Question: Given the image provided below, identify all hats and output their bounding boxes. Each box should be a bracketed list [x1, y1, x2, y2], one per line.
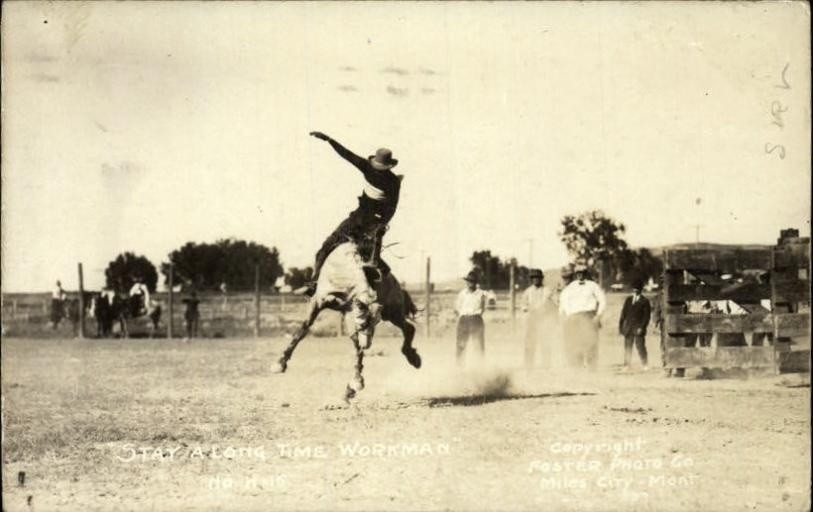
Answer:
[529, 269, 545, 279]
[368, 148, 397, 171]
[632, 278, 642, 289]
[574, 264, 588, 274]
[462, 272, 481, 283]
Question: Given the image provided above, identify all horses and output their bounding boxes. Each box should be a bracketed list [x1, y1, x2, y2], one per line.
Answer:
[268, 235, 425, 400]
[49, 291, 161, 339]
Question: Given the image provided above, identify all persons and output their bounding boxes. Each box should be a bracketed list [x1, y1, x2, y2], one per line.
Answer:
[522, 266, 551, 369]
[558, 264, 606, 369]
[48, 278, 201, 340]
[294, 132, 400, 296]
[617, 279, 650, 366]
[455, 273, 495, 357]
[673, 271, 772, 377]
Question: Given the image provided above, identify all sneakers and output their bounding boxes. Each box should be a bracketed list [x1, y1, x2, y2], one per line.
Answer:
[616, 367, 633, 375]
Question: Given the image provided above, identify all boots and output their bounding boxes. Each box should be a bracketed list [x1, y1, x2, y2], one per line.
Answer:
[293, 271, 318, 296]
